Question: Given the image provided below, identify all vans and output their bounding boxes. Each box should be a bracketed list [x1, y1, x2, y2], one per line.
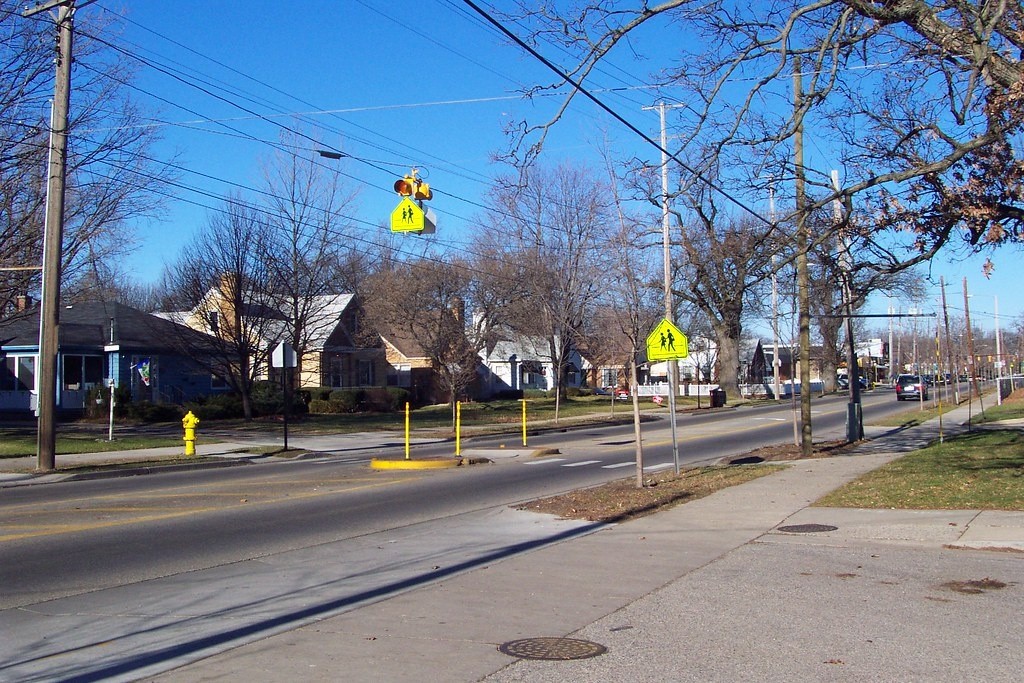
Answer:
[896, 374, 928, 400]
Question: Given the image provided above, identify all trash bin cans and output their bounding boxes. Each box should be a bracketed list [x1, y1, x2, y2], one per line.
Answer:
[710, 390, 727, 408]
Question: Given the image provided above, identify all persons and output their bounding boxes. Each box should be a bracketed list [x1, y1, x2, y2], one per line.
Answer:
[867, 368, 873, 389]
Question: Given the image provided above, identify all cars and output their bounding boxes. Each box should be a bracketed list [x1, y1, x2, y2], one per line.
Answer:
[838, 374, 870, 390]
[924, 372, 987, 385]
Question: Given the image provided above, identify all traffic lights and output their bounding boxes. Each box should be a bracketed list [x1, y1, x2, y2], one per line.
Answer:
[393, 178, 414, 195]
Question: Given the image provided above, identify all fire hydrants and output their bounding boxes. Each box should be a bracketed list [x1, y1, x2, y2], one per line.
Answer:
[181, 411, 201, 456]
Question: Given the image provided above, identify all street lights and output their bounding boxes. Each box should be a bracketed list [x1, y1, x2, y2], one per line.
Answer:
[965, 293, 1002, 405]
